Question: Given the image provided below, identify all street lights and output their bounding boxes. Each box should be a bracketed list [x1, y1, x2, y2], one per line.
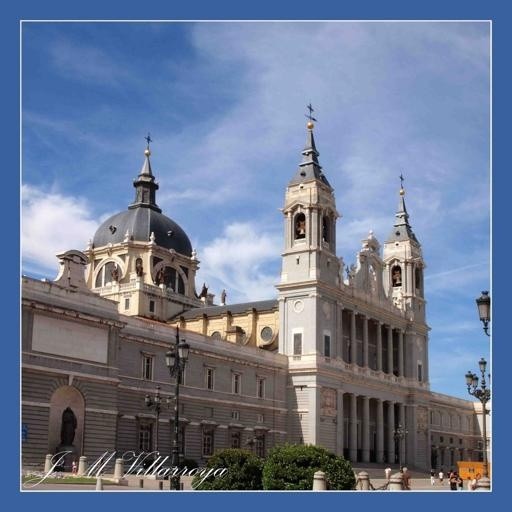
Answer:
[465, 357, 490, 479]
[475, 289, 490, 336]
[166, 325, 189, 490]
[145, 384, 172, 473]
[393, 424, 408, 473]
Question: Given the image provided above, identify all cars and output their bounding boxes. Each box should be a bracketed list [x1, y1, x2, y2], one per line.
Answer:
[124, 455, 165, 475]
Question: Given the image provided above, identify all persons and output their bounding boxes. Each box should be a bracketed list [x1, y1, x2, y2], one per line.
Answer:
[384, 465, 411, 486]
[201, 283, 208, 297]
[110, 266, 119, 281]
[430, 468, 480, 490]
[135, 259, 143, 277]
[221, 290, 226, 303]
[54, 457, 78, 475]
